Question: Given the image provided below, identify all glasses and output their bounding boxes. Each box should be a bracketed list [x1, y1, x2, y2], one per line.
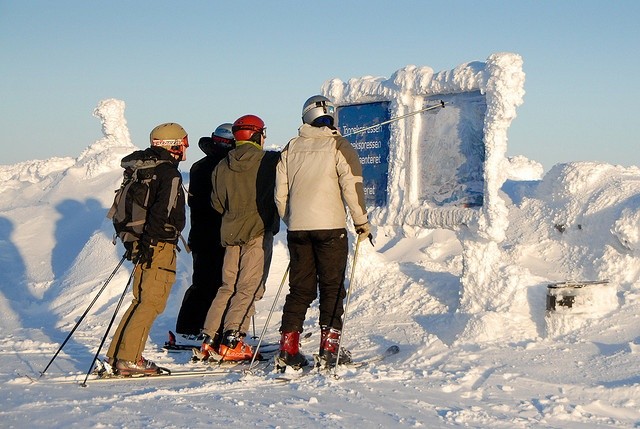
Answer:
[234, 126, 267, 139]
[152, 136, 189, 149]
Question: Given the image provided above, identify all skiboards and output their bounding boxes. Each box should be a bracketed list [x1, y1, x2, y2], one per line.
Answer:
[15, 360, 260, 384]
[235, 345, 400, 388]
[160, 332, 312, 353]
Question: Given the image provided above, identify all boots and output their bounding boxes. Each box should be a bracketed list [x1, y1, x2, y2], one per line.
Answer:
[281, 331, 305, 366]
[319, 326, 351, 362]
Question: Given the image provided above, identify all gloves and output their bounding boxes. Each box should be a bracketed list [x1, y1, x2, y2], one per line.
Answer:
[133, 241, 154, 263]
[354, 223, 370, 242]
[123, 243, 133, 260]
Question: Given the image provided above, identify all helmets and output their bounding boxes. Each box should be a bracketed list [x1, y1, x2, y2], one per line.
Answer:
[302, 95, 334, 124]
[212, 123, 233, 147]
[234, 115, 265, 140]
[149, 123, 189, 161]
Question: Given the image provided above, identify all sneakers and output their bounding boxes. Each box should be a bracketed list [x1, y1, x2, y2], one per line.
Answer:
[116, 357, 160, 375]
[219, 341, 263, 361]
[199, 336, 221, 358]
[175, 333, 205, 347]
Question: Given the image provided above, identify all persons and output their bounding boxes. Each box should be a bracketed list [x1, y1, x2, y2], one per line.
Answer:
[101, 122, 190, 373]
[173, 122, 237, 348]
[200, 114, 282, 359]
[273, 95, 371, 372]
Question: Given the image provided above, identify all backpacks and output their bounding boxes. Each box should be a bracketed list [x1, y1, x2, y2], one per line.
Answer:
[107, 150, 174, 242]
